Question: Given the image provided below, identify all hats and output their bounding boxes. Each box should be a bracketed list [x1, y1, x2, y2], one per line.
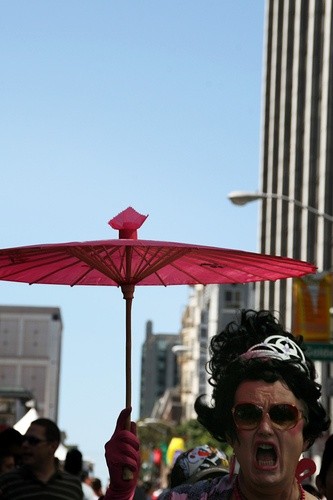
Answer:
[171, 446, 230, 486]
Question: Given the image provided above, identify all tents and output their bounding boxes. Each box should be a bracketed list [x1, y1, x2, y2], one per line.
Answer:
[13, 408, 68, 462]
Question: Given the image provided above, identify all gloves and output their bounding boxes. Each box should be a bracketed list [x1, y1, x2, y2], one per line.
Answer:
[104, 407, 141, 500]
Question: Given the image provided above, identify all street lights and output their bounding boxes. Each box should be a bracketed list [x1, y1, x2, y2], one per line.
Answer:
[171, 345, 189, 425]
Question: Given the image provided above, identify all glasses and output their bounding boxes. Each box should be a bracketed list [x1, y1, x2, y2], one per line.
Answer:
[232, 403, 305, 431]
[20, 435, 49, 447]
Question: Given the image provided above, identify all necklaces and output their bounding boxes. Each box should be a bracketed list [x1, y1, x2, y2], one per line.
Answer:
[234, 480, 305, 500]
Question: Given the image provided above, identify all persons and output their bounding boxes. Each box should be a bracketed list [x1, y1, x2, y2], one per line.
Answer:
[168, 444, 230, 489]
[101, 309, 332, 500]
[91, 478, 104, 497]
[315, 434, 333, 500]
[64, 446, 100, 500]
[0, 418, 85, 500]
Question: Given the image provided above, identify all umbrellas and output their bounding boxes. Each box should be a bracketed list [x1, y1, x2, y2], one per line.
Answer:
[0, 206, 318, 482]
